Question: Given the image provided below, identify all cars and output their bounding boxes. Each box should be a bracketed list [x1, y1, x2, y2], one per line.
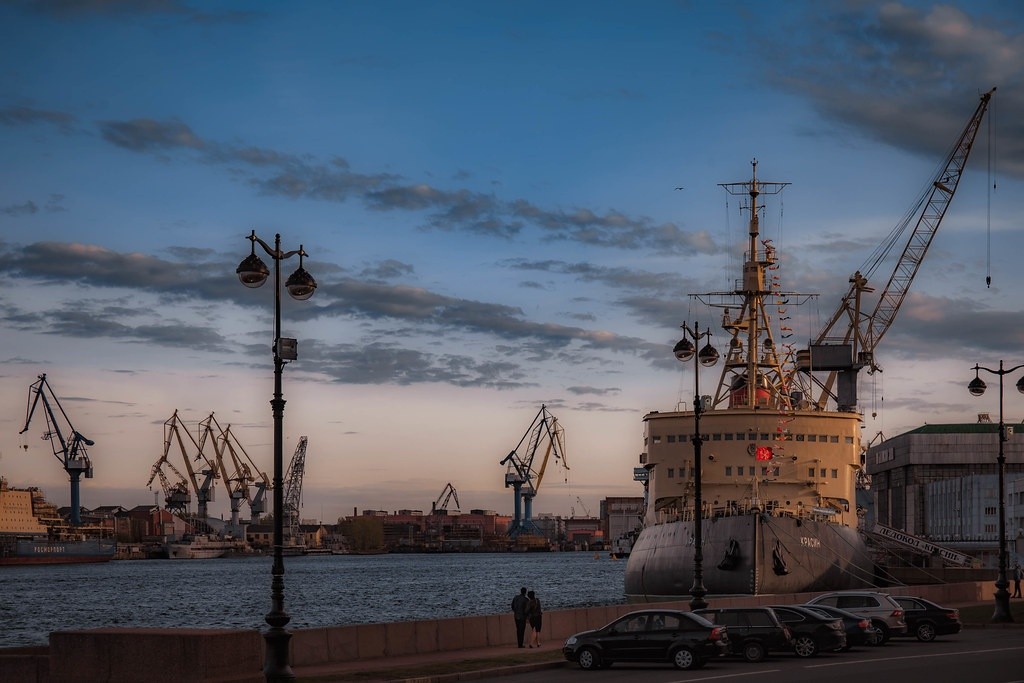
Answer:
[795, 604, 877, 654]
[563, 609, 732, 670]
[890, 594, 962, 643]
[763, 604, 848, 658]
[689, 607, 791, 662]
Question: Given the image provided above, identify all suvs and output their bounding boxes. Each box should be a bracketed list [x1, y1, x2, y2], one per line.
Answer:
[805, 592, 908, 646]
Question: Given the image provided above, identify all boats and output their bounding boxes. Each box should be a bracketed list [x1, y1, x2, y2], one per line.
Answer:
[268, 545, 309, 558]
[0, 528, 118, 565]
[619, 150, 886, 600]
[329, 547, 351, 556]
[609, 537, 633, 557]
[167, 534, 245, 559]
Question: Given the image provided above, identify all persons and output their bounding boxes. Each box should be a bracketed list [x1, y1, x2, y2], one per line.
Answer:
[524, 591, 543, 648]
[626, 617, 646, 632]
[511, 587, 529, 647]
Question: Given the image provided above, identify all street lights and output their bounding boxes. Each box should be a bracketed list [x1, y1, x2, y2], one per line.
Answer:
[968, 355, 1024, 624]
[233, 228, 318, 682]
[671, 317, 722, 614]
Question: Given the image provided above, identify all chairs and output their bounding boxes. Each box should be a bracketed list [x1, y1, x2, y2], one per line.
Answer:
[647, 621, 658, 630]
[656, 619, 664, 629]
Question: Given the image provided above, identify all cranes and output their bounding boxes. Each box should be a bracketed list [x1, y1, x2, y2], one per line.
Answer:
[145, 406, 316, 549]
[499, 400, 577, 552]
[421, 482, 461, 554]
[818, 87, 1005, 587]
[17, 370, 96, 530]
